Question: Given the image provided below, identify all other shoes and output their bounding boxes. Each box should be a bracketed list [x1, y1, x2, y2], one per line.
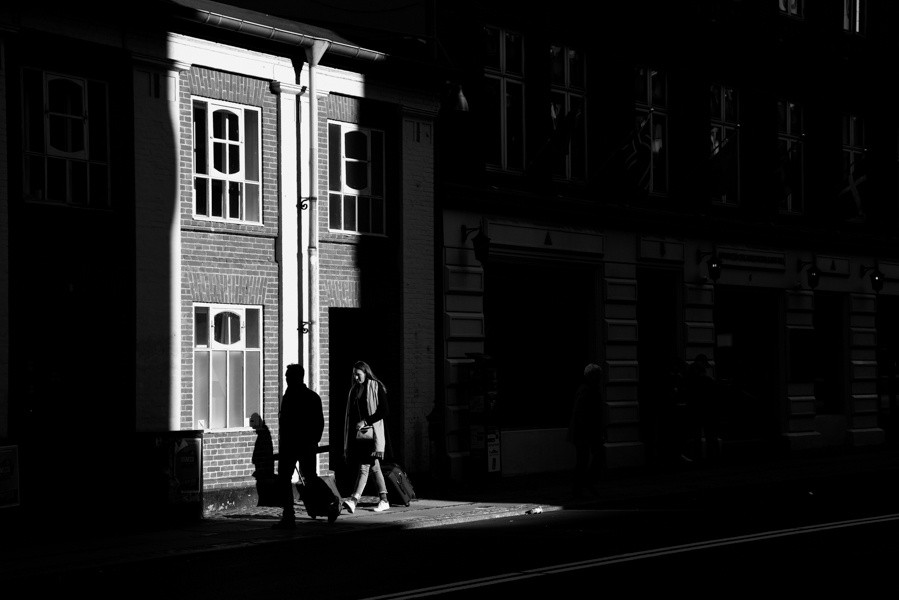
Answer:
[344, 500, 355, 513]
[374, 501, 390, 511]
[271, 520, 297, 529]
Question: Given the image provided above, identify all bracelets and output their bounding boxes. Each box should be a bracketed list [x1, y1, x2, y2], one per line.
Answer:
[364, 420, 368, 426]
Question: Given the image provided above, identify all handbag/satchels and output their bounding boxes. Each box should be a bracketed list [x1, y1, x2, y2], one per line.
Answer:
[356, 426, 374, 440]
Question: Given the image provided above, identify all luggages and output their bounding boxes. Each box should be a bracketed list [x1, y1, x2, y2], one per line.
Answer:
[372, 464, 416, 506]
[295, 466, 343, 519]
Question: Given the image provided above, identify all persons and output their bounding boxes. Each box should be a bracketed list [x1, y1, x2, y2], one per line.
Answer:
[570, 363, 608, 489]
[343, 361, 390, 514]
[679, 354, 725, 462]
[271, 364, 341, 530]
